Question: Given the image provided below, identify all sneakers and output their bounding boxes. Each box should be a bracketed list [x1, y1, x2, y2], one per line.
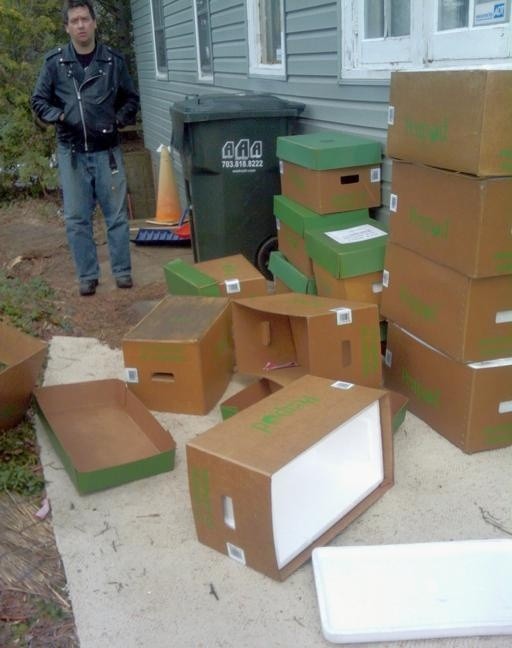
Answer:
[116, 275, 133, 288]
[80, 279, 99, 296]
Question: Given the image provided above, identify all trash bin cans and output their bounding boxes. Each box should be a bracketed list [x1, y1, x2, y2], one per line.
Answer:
[170, 90, 306, 282]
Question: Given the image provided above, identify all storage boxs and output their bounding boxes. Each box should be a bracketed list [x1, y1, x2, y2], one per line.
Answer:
[222, 70, 512, 456]
[2, 322, 50, 428]
[122, 297, 232, 416]
[165, 254, 266, 298]
[186, 374, 396, 581]
[34, 377, 177, 496]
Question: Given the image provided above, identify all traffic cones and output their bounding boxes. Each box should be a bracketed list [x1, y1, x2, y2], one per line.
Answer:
[144, 145, 189, 226]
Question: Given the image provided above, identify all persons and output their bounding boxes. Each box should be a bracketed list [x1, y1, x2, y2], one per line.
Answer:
[30, 0, 140, 295]
